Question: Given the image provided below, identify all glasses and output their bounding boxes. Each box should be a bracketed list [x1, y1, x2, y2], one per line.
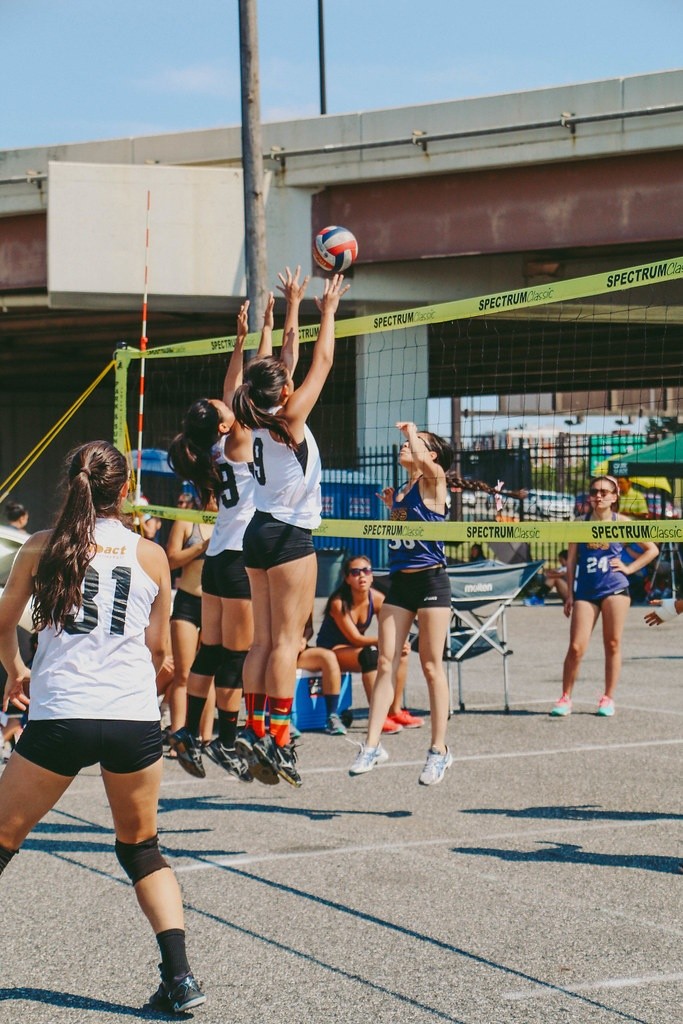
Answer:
[177, 501, 186, 505]
[590, 489, 612, 497]
[407, 436, 432, 452]
[348, 567, 372, 576]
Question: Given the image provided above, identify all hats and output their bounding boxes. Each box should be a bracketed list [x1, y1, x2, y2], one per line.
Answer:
[131, 496, 151, 525]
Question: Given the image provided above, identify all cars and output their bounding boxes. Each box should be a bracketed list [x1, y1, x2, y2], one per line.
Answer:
[645, 494, 679, 520]
[458, 490, 476, 508]
[574, 494, 590, 513]
[487, 490, 575, 518]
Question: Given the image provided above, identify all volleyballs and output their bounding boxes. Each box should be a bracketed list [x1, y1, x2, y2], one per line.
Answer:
[313, 225, 358, 273]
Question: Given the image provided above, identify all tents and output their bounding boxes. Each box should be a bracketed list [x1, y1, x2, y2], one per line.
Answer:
[606, 432, 683, 480]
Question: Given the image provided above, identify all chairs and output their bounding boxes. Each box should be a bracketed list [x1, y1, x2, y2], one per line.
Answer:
[372, 559, 547, 718]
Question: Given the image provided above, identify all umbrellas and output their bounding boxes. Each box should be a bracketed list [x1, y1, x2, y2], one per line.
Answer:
[595, 453, 672, 492]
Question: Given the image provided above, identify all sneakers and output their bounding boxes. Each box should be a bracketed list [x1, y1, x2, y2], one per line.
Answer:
[234, 728, 280, 785]
[148, 962, 207, 1012]
[552, 692, 572, 716]
[289, 722, 301, 739]
[381, 717, 403, 734]
[344, 737, 389, 775]
[168, 727, 206, 778]
[387, 710, 425, 729]
[253, 734, 303, 788]
[597, 695, 615, 716]
[203, 737, 254, 783]
[418, 744, 453, 787]
[325, 713, 347, 735]
[523, 595, 544, 607]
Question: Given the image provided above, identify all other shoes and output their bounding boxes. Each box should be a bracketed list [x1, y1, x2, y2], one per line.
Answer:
[162, 723, 172, 743]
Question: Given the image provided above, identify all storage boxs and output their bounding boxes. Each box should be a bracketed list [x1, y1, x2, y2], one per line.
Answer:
[290, 668, 352, 735]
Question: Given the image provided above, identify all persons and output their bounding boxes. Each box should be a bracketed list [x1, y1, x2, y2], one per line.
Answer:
[469, 544, 485, 562]
[0, 265, 348, 788]
[349, 422, 454, 785]
[521, 497, 683, 627]
[546, 481, 660, 717]
[0, 439, 209, 1013]
[320, 555, 425, 734]
